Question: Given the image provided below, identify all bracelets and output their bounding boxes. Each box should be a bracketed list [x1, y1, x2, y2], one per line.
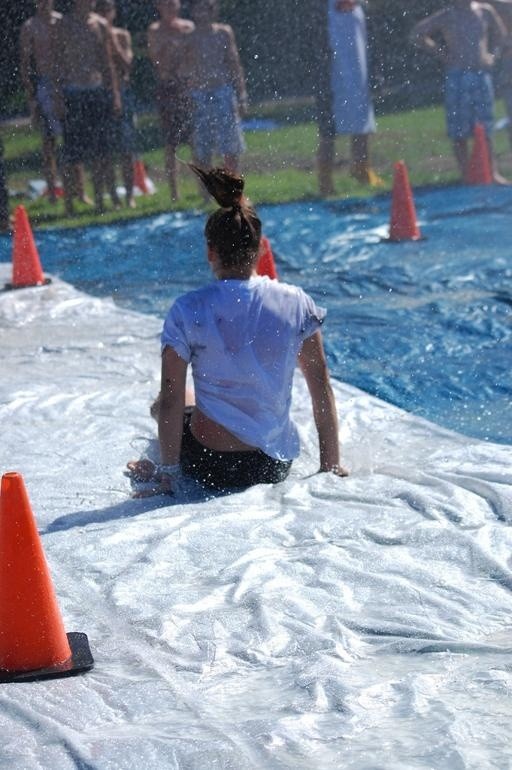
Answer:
[158, 463, 183, 475]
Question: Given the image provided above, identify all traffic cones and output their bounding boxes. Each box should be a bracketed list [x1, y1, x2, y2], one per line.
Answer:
[4, 203, 54, 290]
[0, 468, 95, 686]
[457, 124, 492, 185]
[378, 160, 429, 242]
[255, 234, 277, 280]
[40, 157, 64, 198]
[135, 161, 150, 196]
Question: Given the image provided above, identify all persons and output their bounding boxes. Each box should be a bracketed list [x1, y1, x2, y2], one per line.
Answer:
[18, 0, 137, 217]
[327, 0, 385, 187]
[126, 156, 350, 498]
[146, 0, 249, 210]
[409, 0, 512, 186]
